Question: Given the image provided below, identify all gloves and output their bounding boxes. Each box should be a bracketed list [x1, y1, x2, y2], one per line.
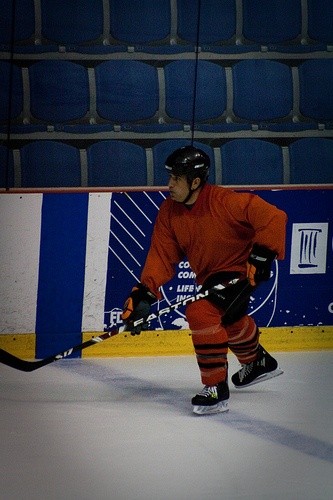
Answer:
[245, 242, 277, 286]
[121, 283, 158, 336]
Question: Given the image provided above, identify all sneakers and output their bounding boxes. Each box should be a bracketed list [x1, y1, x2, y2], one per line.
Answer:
[192, 380, 231, 415]
[231, 345, 284, 388]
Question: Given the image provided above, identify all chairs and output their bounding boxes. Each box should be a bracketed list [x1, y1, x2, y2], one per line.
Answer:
[0, 0, 333, 187]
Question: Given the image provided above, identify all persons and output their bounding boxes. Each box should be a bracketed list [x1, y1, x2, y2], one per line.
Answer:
[122, 145, 287, 406]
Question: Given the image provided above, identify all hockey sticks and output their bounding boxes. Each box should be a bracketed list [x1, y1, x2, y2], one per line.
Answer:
[0, 269, 276, 372]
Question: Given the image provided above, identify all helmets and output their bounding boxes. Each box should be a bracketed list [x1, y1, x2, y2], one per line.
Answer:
[166, 145, 211, 184]
